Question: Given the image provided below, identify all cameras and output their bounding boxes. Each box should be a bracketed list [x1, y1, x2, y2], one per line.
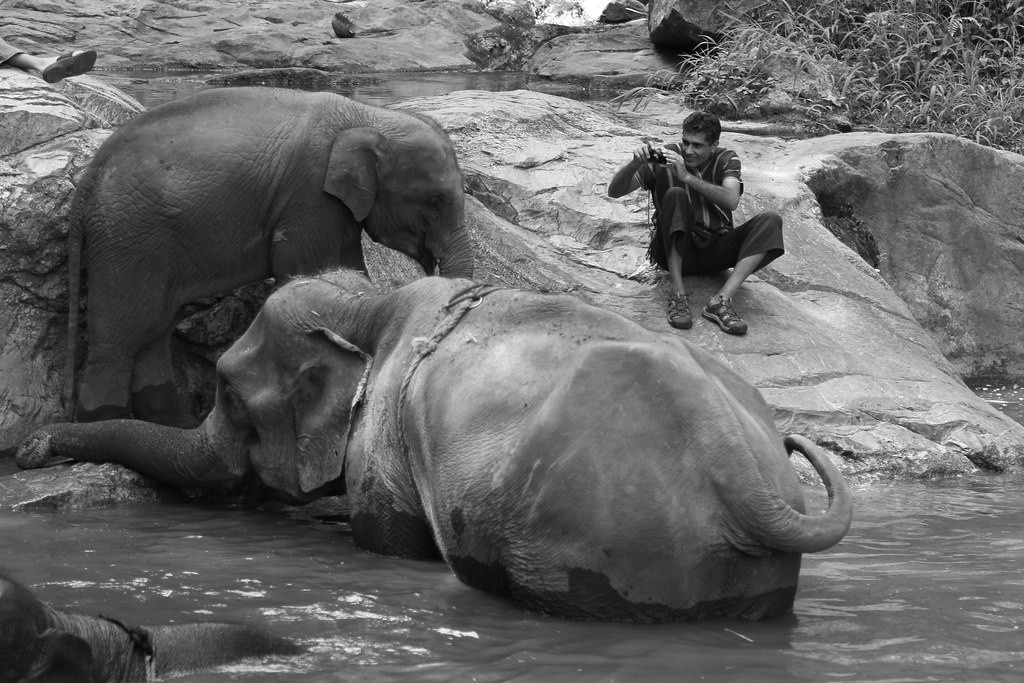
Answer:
[646, 143, 682, 164]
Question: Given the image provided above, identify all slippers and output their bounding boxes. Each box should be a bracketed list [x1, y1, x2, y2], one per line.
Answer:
[43, 49, 97, 83]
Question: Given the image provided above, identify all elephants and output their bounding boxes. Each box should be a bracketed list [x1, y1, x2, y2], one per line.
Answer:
[17, 85, 855, 624]
[0, 569, 310, 683]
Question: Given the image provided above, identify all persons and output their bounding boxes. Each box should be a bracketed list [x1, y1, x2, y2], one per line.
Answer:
[608, 112, 785, 336]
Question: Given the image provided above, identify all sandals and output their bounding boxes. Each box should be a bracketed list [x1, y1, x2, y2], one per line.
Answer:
[701, 296, 747, 334]
[667, 292, 692, 329]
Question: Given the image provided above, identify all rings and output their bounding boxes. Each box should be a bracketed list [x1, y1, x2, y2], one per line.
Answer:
[676, 157, 679, 160]
